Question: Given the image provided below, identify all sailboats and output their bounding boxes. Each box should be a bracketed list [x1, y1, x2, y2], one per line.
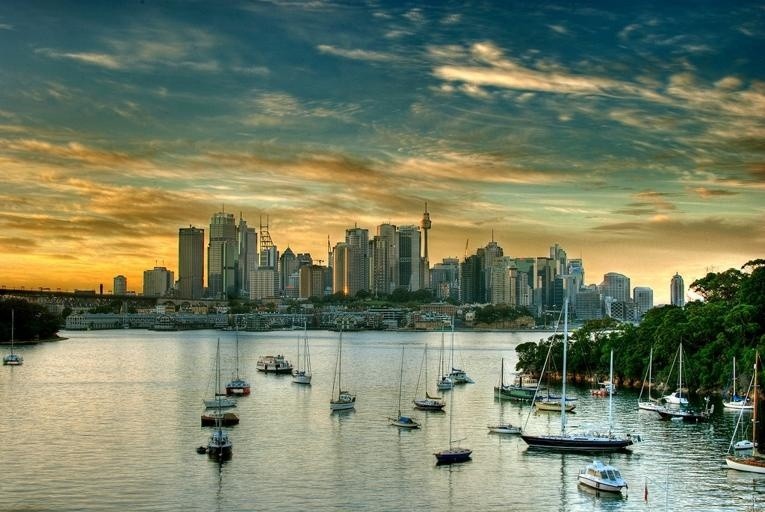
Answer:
[3, 309, 23, 365]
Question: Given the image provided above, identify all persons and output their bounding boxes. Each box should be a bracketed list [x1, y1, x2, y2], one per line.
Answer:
[600, 385, 607, 391]
[756, 387, 765, 455]
